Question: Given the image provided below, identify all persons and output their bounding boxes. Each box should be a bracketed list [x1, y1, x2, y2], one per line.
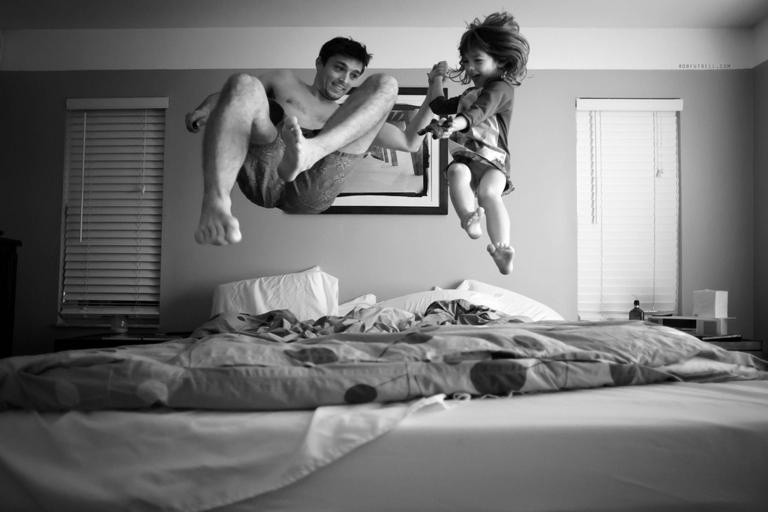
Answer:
[184, 36, 450, 245]
[428, 13, 530, 275]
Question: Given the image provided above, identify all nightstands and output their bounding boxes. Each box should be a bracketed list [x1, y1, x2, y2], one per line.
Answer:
[648, 316, 764, 352]
[93, 322, 182, 347]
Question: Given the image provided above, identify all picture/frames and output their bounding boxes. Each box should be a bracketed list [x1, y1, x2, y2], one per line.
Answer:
[326, 85, 449, 220]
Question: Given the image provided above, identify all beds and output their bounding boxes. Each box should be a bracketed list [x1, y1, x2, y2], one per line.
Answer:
[0, 317, 768, 512]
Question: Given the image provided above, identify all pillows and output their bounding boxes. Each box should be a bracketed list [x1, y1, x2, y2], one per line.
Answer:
[212, 270, 341, 338]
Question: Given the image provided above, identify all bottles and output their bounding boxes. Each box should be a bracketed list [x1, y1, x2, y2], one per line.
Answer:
[629, 300, 644, 322]
